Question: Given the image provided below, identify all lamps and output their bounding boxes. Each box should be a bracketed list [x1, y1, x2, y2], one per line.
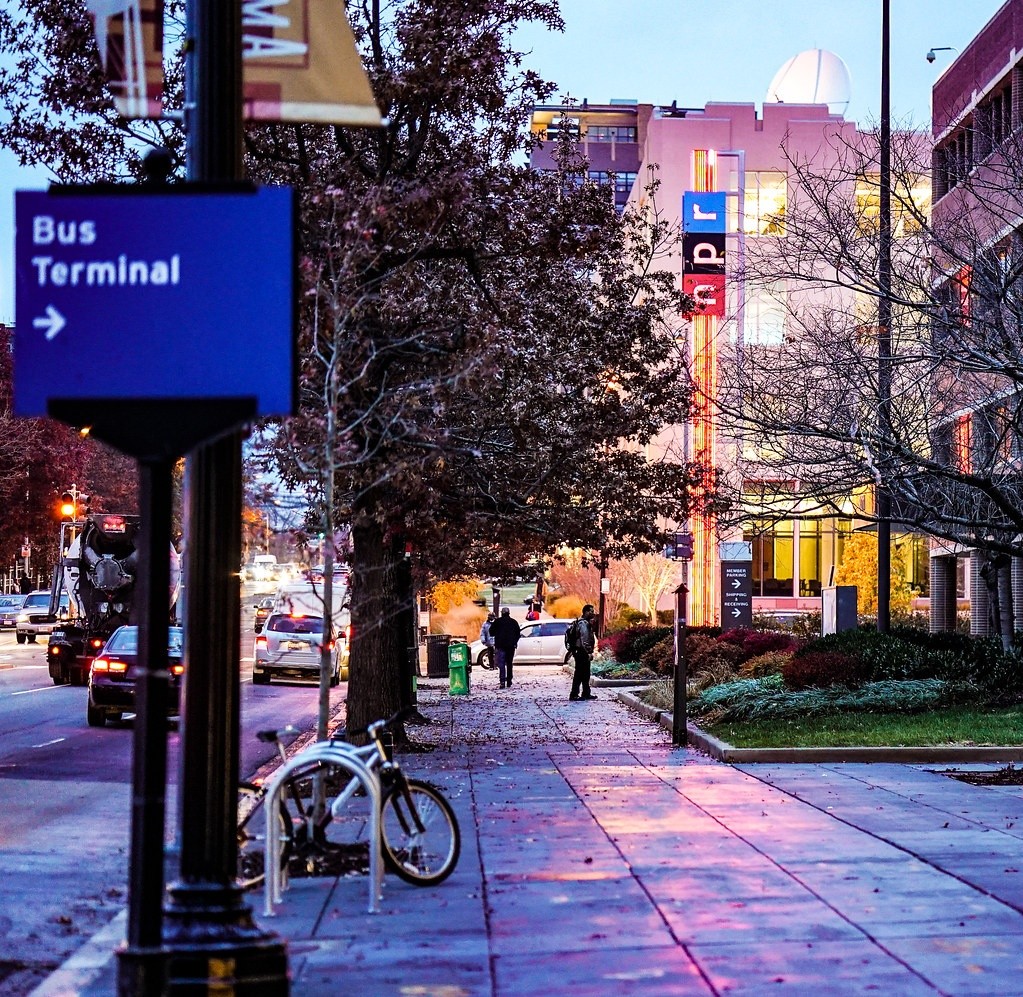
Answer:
[927, 47, 960, 63]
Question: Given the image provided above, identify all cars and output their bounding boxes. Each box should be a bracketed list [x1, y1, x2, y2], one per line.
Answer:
[469, 619, 598, 669]
[0, 593, 30, 629]
[250, 612, 347, 687]
[243, 554, 353, 668]
[86, 624, 184, 728]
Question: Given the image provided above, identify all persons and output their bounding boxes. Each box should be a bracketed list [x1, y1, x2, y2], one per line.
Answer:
[520, 626, 540, 637]
[569, 605, 598, 701]
[481, 612, 497, 670]
[528, 597, 542, 620]
[13, 570, 31, 594]
[489, 608, 520, 689]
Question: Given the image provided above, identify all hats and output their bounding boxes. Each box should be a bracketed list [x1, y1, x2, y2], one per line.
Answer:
[486, 612, 495, 620]
[501, 608, 510, 614]
[532, 596, 540, 604]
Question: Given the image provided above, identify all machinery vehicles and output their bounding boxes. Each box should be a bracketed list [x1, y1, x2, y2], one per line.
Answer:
[44, 513, 185, 688]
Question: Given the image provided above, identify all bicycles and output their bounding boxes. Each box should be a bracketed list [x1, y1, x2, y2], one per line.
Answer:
[236, 707, 462, 888]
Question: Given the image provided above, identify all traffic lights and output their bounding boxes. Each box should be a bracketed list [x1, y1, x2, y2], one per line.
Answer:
[60, 491, 75, 516]
[77, 490, 91, 516]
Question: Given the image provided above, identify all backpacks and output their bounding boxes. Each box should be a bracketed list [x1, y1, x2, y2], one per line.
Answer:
[564, 618, 589, 654]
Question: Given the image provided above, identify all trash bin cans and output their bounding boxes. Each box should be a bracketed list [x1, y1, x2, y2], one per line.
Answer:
[422, 633, 452, 679]
[447, 640, 473, 695]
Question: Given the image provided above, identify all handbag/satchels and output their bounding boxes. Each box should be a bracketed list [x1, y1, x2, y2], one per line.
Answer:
[525, 612, 535, 621]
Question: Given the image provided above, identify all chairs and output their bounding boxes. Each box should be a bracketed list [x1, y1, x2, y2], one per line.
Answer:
[125, 642, 137, 648]
[276, 621, 295, 630]
[266, 601, 272, 606]
[298, 622, 317, 631]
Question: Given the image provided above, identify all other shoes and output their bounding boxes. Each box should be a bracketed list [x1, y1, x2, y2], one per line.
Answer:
[500, 683, 505, 689]
[581, 694, 597, 699]
[494, 667, 498, 670]
[489, 667, 493, 671]
[507, 681, 512, 687]
[570, 695, 585, 701]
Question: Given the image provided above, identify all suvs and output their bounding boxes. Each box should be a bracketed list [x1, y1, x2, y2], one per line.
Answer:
[14, 587, 75, 644]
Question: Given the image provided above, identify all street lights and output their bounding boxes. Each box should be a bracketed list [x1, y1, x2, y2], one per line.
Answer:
[267, 499, 281, 555]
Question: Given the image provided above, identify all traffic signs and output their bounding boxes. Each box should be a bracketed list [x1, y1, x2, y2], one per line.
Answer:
[5, 182, 302, 424]
